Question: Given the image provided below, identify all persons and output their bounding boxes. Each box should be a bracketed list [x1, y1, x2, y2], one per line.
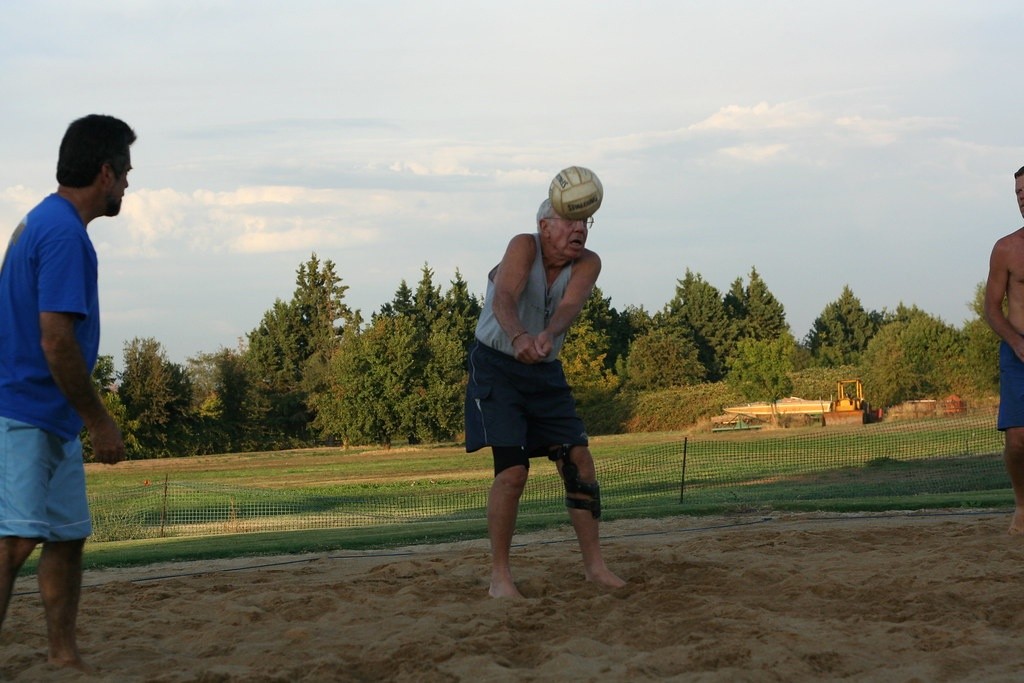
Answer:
[0, 114, 138, 676]
[464, 199, 626, 599]
[984, 166, 1024, 536]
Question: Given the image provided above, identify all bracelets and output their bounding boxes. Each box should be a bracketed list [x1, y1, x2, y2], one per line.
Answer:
[511, 330, 529, 346]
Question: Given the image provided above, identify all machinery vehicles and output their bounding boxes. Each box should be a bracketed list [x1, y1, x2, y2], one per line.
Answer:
[822, 379, 872, 426]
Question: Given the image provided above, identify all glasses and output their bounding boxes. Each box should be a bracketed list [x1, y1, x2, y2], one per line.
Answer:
[543, 216, 594, 228]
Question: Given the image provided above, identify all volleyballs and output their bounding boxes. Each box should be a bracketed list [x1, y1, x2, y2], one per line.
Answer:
[547, 165, 605, 219]
